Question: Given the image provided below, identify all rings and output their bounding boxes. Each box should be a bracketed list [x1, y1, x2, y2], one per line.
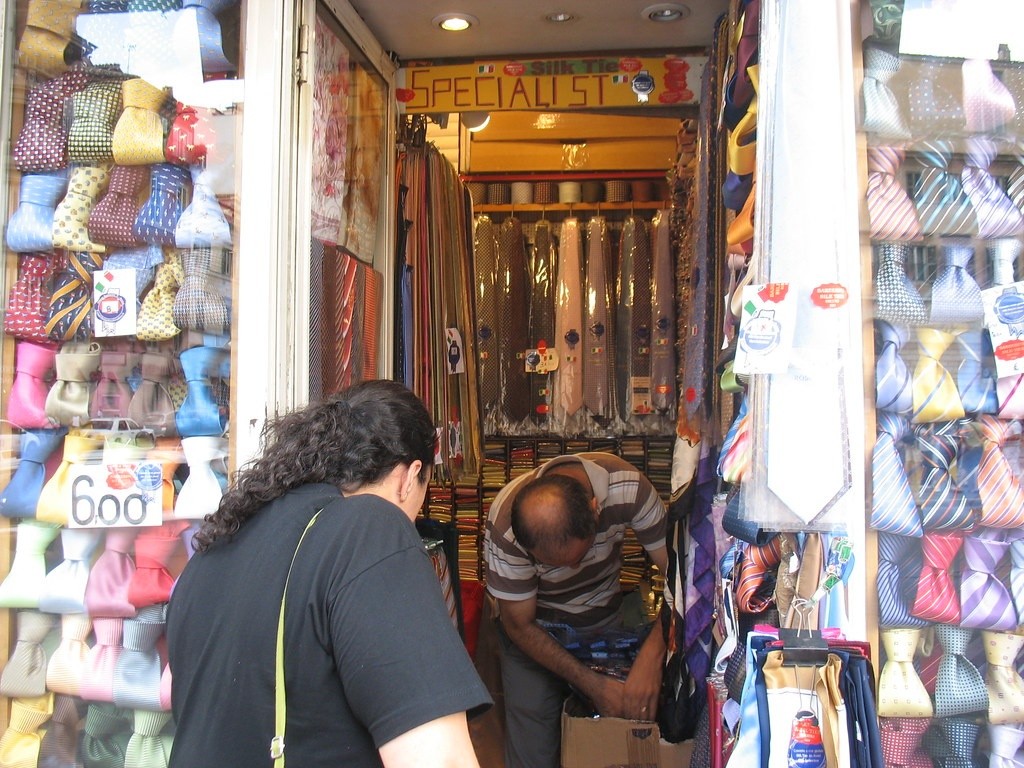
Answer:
[640, 706, 648, 712]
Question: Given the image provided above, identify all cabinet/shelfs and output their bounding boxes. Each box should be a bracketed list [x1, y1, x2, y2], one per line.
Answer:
[415, 171, 676, 602]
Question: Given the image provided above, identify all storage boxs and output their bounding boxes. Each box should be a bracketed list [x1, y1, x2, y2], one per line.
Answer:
[559, 688, 694, 768]
[469, 111, 685, 173]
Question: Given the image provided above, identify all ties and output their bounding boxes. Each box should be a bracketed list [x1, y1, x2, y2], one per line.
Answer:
[399, 147, 466, 474]
[310, 235, 381, 400]
[715, 0, 852, 739]
[862, 48, 1024, 768]
[0, 1, 233, 766]
[473, 180, 676, 439]
[682, 58, 714, 421]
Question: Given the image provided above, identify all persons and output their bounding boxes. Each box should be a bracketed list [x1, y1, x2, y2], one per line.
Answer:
[482, 448, 678, 768]
[160, 379, 496, 768]
